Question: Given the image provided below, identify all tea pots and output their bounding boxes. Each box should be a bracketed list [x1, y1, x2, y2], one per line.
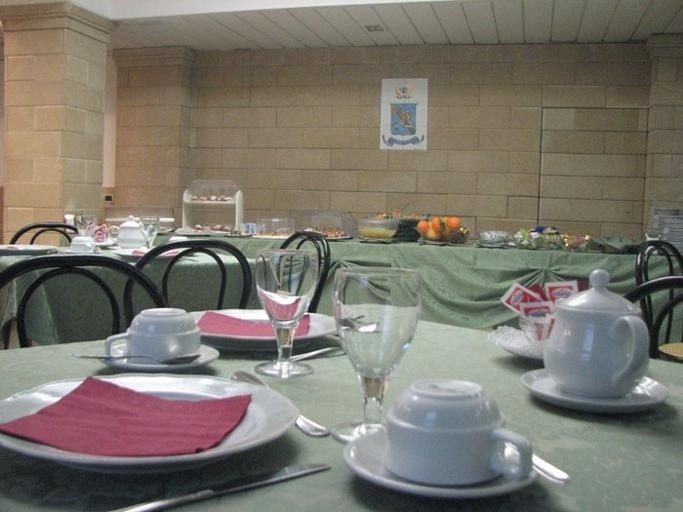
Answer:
[117, 215, 153, 250]
[541, 269, 650, 400]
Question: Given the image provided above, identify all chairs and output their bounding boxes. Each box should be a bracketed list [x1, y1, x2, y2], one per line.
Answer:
[276, 230, 331, 313]
[0, 254, 168, 348]
[8, 221, 80, 246]
[635, 239, 683, 363]
[622, 275, 683, 363]
[123, 238, 252, 329]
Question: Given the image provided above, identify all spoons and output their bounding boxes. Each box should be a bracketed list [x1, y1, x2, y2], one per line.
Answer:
[70, 352, 200, 365]
[289, 322, 381, 364]
[231, 371, 331, 437]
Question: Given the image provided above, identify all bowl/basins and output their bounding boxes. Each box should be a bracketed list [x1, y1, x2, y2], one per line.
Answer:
[519, 315, 546, 347]
[391, 216, 423, 242]
[357, 217, 397, 239]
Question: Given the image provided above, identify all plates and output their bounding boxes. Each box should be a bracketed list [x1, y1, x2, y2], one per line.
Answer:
[0, 372, 299, 473]
[190, 308, 337, 352]
[417, 237, 465, 248]
[341, 433, 539, 500]
[487, 324, 547, 362]
[113, 251, 185, 262]
[252, 234, 291, 241]
[95, 345, 220, 375]
[474, 240, 505, 248]
[325, 236, 350, 243]
[519, 367, 669, 413]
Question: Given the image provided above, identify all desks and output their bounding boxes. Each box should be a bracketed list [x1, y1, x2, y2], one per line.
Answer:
[0, 319, 682, 512]
[105, 224, 683, 332]
[1, 243, 272, 350]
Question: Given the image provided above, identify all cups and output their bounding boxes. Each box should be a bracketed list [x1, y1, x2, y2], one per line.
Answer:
[253, 250, 321, 379]
[103, 307, 201, 364]
[332, 267, 421, 447]
[69, 236, 95, 251]
[383, 378, 534, 485]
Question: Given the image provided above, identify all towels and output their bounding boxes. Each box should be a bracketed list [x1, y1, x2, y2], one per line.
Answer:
[0, 371, 252, 455]
[197, 309, 311, 338]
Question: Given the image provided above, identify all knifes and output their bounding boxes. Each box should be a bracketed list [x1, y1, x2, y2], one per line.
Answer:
[96, 460, 331, 512]
